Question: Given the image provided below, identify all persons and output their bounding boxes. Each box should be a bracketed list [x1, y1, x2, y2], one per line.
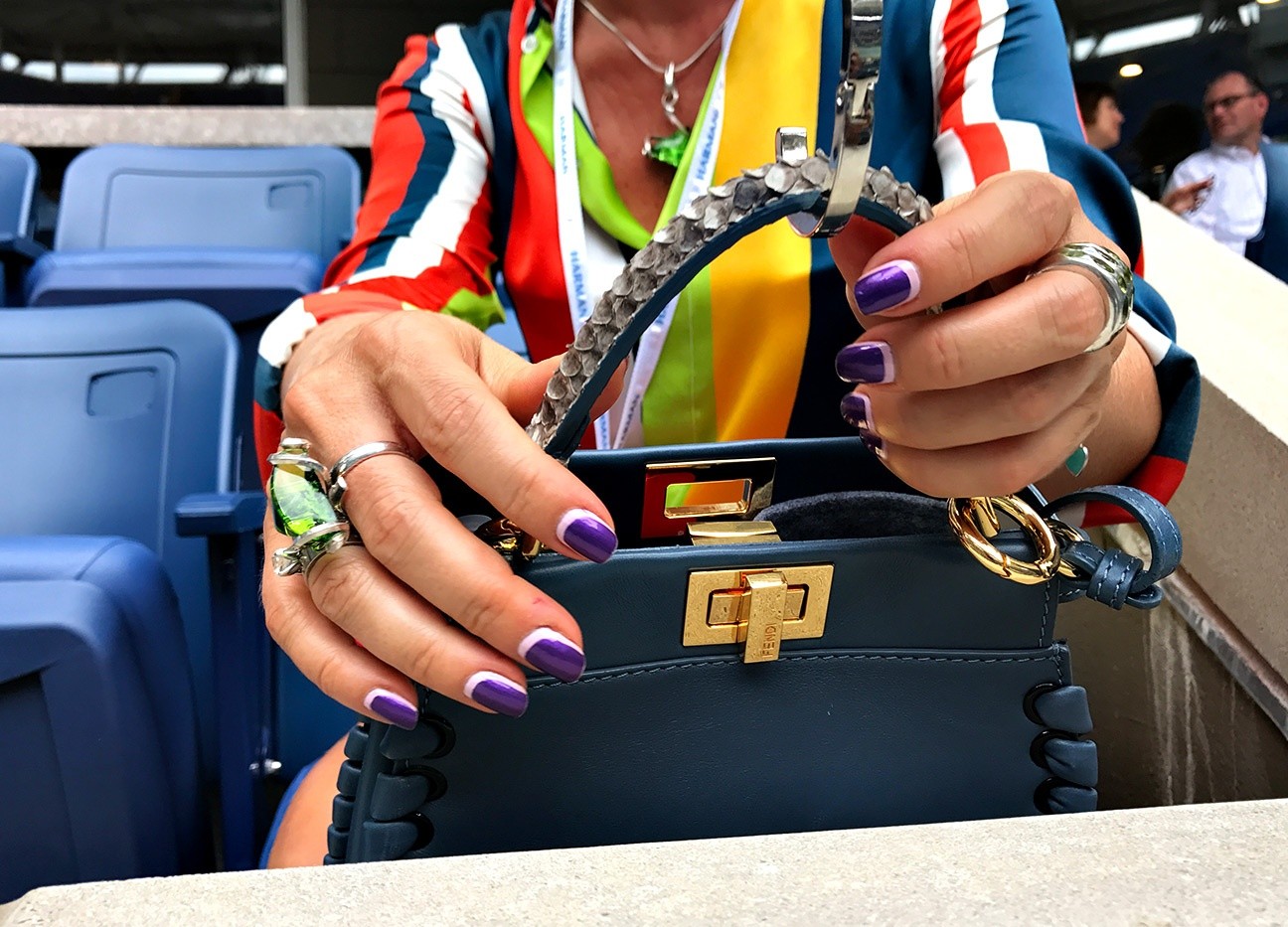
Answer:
[259, 0, 1200, 870]
[1077, 69, 1288, 286]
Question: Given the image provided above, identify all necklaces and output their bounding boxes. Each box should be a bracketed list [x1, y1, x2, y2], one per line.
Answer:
[581, 0, 726, 187]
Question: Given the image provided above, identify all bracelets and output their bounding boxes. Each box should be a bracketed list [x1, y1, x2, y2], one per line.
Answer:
[1066, 445, 1088, 477]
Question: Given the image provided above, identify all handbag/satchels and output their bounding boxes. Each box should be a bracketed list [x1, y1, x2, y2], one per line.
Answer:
[319, 77, 1182, 861]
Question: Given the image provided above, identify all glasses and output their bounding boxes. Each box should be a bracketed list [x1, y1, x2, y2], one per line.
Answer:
[1196, 91, 1259, 114]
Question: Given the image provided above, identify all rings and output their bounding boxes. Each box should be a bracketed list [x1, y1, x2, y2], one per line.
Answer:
[267, 437, 421, 589]
[1025, 243, 1133, 352]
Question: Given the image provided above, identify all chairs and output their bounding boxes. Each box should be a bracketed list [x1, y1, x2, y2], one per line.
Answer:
[0, 141, 361, 903]
